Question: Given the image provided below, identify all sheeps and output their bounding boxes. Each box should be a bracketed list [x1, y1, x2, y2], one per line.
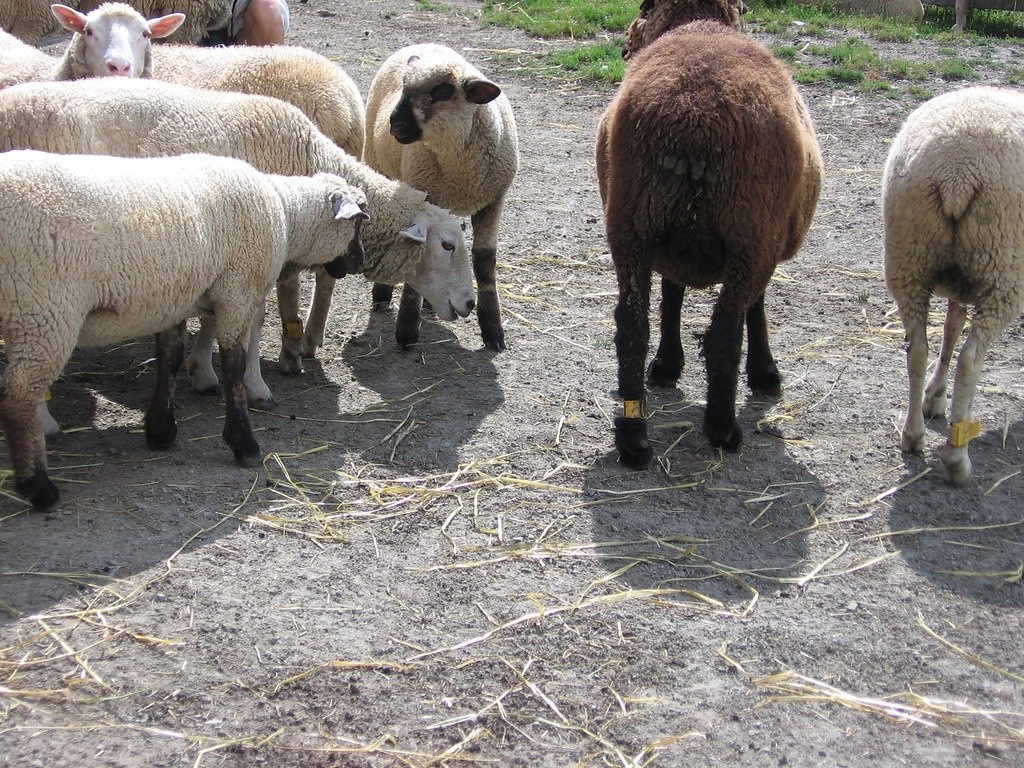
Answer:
[363, 41, 521, 356]
[879, 83, 1024, 491]
[593, 0, 826, 471]
[0, 0, 480, 514]
